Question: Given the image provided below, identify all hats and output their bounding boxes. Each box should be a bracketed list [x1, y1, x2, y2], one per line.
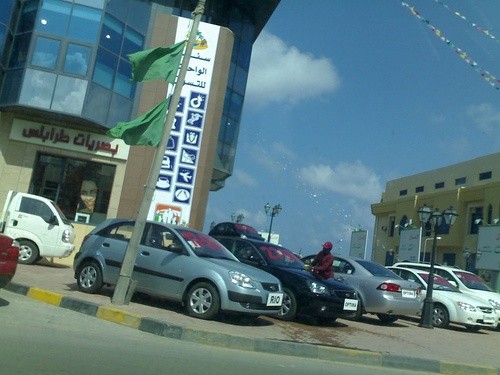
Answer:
[321, 241, 332, 249]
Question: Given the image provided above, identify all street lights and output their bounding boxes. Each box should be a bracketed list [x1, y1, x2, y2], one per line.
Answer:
[264, 202, 283, 241]
[418, 204, 460, 326]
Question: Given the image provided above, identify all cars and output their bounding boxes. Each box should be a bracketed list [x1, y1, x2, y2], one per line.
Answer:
[301, 254, 424, 324]
[208, 222, 264, 240]
[72, 217, 286, 320]
[385, 266, 498, 331]
[212, 235, 364, 325]
[0, 234, 21, 277]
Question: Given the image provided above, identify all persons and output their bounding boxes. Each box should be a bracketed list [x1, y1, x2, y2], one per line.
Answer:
[309, 241, 335, 279]
[80, 180, 98, 212]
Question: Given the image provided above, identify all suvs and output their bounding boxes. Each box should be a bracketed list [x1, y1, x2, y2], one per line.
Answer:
[391, 261, 500, 323]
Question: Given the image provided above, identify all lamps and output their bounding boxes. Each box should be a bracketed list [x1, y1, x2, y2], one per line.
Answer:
[405, 223, 409, 228]
[474, 218, 488, 225]
[395, 224, 404, 230]
[493, 218, 499, 224]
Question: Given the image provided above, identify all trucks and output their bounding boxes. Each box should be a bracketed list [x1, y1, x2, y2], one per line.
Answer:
[0, 189, 77, 266]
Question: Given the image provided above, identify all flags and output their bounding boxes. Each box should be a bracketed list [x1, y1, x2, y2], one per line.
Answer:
[127, 40, 186, 86]
[106, 96, 172, 148]
